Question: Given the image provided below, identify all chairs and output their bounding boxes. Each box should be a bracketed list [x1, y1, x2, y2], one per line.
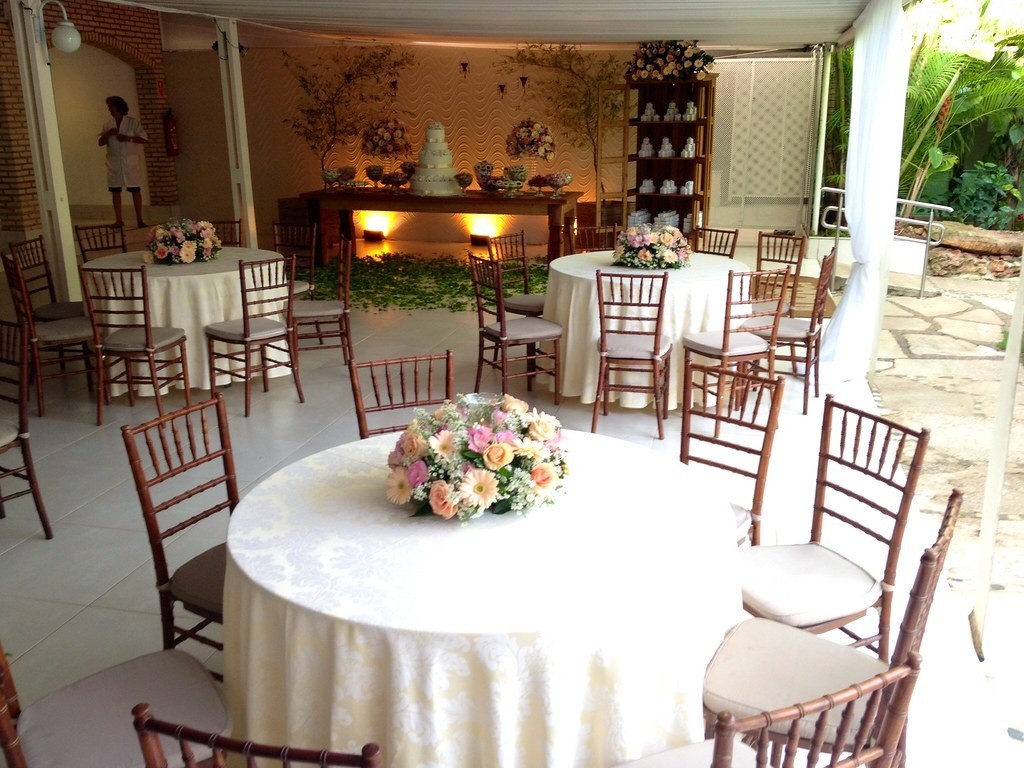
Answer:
[0, 221, 966, 768]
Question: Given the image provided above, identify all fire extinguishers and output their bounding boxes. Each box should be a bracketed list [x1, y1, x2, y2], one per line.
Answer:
[165, 108, 179, 156]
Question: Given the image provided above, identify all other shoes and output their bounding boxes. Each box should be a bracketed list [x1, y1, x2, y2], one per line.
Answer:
[109, 223, 125, 229]
[138, 222, 148, 228]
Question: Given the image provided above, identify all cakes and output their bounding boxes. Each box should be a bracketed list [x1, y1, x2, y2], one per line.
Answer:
[410, 122, 461, 194]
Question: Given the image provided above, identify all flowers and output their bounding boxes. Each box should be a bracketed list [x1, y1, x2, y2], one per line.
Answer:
[143, 218, 222, 264]
[501, 116, 558, 162]
[383, 387, 573, 526]
[361, 112, 412, 161]
[607, 221, 694, 270]
[621, 32, 718, 82]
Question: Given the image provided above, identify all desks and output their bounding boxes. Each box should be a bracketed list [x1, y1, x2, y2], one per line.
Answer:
[299, 187, 584, 283]
[83, 243, 286, 397]
[530, 250, 751, 405]
[225, 413, 741, 768]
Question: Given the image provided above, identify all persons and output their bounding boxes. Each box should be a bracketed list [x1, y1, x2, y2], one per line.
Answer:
[96, 96, 149, 228]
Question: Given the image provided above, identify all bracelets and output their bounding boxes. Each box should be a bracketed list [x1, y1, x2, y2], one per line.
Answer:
[130, 137, 132, 142]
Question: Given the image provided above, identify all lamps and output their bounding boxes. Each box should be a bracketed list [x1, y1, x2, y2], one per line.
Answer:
[470, 234, 490, 246]
[31, 0, 81, 64]
[364, 230, 383, 241]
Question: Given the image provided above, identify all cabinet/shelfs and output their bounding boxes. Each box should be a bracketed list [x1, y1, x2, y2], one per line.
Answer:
[599, 69, 720, 250]
